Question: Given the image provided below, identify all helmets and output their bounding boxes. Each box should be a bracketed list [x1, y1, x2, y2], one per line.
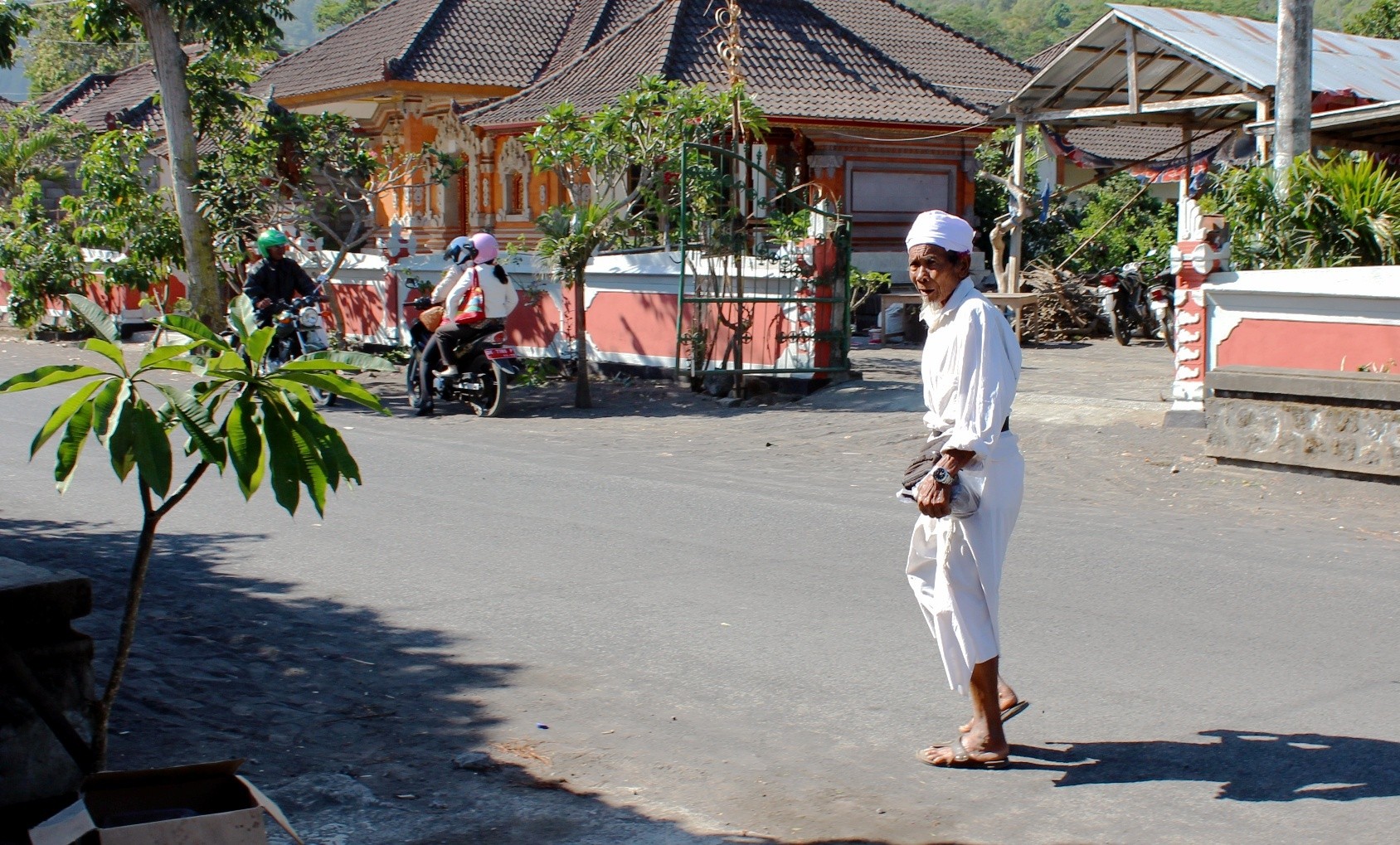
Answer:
[464, 232, 499, 264]
[443, 236, 473, 265]
[257, 230, 288, 258]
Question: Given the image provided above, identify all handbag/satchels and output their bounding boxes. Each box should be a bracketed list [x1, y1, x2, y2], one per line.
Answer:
[454, 266, 486, 327]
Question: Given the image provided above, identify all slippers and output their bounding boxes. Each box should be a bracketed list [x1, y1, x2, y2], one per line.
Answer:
[959, 700, 1029, 733]
[916, 735, 1009, 769]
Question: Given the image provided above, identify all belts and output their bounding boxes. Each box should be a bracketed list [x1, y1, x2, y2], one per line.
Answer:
[1001, 416, 1009, 432]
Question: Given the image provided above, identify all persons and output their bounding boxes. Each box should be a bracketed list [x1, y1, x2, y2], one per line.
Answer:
[242, 229, 330, 375]
[896, 209, 1030, 771]
[432, 232, 519, 379]
[413, 236, 477, 417]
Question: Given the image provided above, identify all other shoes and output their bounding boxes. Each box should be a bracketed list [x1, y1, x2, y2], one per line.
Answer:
[432, 365, 459, 379]
[415, 401, 434, 416]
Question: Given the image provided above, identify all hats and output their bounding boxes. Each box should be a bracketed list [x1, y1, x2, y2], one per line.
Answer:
[905, 210, 976, 255]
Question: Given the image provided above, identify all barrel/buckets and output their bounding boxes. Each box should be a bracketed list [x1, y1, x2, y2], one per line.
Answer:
[868, 329, 882, 345]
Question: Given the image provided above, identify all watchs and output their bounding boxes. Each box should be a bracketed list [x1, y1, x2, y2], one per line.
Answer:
[930, 465, 955, 486]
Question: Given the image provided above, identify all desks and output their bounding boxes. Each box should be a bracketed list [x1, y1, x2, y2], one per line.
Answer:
[878, 293, 1039, 346]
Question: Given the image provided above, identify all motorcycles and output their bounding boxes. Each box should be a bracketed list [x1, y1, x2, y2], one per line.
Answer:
[229, 274, 339, 407]
[401, 277, 520, 419]
[1094, 248, 1176, 354]
